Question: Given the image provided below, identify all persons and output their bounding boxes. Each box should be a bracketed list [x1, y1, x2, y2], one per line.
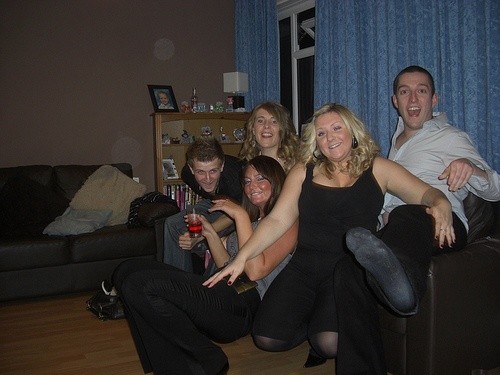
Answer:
[335, 66, 500, 375]
[202, 102, 456, 355]
[158, 92, 174, 109]
[166, 139, 248, 291]
[240, 102, 299, 174]
[112, 156, 299, 375]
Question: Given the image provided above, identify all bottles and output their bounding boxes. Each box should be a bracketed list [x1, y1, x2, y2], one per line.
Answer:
[189, 87, 198, 112]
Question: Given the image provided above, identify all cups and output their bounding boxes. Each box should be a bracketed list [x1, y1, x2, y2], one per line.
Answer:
[186, 204, 202, 238]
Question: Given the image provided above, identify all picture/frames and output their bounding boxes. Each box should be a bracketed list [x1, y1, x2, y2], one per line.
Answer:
[148, 84, 179, 112]
[163, 159, 180, 179]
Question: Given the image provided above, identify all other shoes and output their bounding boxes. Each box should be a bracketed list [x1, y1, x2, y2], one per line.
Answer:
[303, 347, 326, 367]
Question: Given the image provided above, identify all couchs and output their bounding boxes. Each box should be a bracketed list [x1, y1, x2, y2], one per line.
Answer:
[157, 193, 500, 375]
[0, 162, 178, 302]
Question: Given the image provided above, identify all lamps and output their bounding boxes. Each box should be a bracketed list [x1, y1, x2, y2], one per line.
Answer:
[223, 72, 248, 108]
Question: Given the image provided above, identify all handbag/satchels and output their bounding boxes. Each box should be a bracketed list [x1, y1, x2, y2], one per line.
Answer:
[128, 190, 181, 228]
[87, 281, 124, 320]
[223, 270, 258, 294]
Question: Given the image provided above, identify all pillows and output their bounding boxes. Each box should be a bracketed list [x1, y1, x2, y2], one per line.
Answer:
[70, 166, 145, 225]
[44, 208, 112, 236]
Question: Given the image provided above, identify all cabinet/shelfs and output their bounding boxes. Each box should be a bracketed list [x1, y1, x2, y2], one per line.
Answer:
[149, 111, 253, 211]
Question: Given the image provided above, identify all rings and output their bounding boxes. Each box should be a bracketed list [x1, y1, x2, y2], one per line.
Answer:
[440, 229, 446, 231]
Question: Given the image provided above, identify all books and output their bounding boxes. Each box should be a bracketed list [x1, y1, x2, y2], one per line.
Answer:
[163, 184, 198, 211]
